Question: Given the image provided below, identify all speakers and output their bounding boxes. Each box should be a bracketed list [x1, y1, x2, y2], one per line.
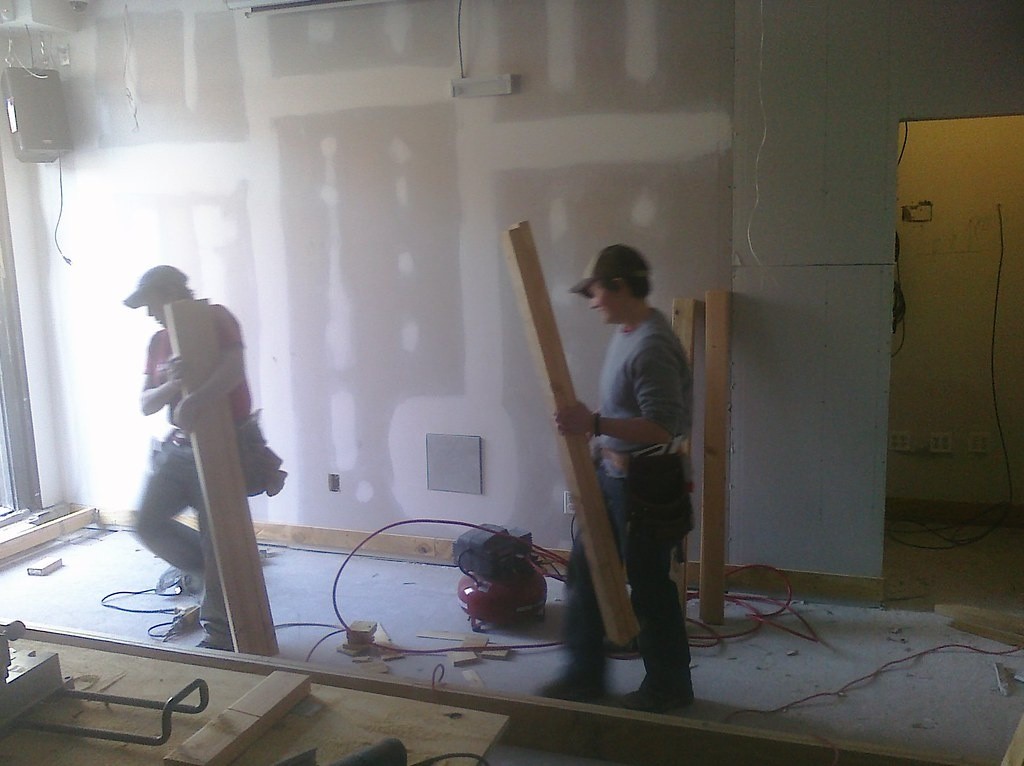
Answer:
[0, 67, 73, 163]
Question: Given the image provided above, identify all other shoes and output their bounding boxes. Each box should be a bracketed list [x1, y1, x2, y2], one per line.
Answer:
[623, 688, 694, 711]
[197, 641, 234, 652]
[536, 678, 602, 704]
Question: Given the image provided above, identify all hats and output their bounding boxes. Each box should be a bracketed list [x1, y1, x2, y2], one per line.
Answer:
[568, 244, 650, 300]
[123, 265, 188, 309]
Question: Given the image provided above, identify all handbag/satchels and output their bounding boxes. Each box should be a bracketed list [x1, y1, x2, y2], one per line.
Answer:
[631, 454, 694, 544]
[237, 407, 288, 497]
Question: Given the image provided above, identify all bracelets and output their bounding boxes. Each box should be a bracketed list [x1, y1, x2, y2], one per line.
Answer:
[595, 413, 601, 437]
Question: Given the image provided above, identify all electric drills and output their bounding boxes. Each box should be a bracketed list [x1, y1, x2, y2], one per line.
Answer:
[162, 605, 201, 639]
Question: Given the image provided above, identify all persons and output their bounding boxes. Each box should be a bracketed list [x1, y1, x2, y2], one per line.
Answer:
[537, 245, 695, 711]
[124, 265, 250, 650]
